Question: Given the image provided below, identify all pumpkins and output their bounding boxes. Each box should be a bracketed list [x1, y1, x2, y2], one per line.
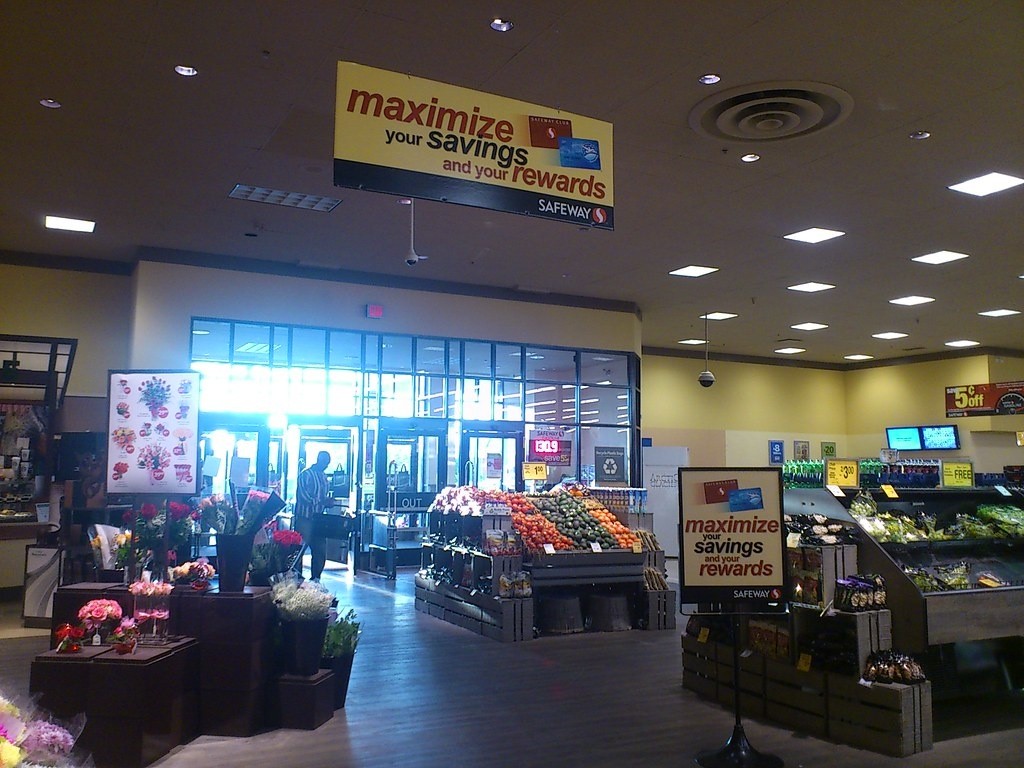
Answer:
[476, 489, 641, 552]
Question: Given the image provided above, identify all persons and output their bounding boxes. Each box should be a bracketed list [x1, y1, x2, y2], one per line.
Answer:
[292, 451, 335, 580]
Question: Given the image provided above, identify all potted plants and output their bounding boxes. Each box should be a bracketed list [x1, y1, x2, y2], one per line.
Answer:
[319, 609, 361, 710]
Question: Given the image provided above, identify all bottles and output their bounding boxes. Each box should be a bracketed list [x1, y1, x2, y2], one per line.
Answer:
[782, 459, 823, 489]
[860, 459, 940, 487]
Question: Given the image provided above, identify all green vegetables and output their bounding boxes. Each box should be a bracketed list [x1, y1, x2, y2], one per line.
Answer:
[901, 563, 970, 593]
[846, 496, 1024, 544]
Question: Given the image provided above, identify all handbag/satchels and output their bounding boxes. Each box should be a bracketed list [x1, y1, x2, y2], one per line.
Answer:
[334, 464, 344, 485]
[398, 464, 409, 485]
[267, 463, 275, 480]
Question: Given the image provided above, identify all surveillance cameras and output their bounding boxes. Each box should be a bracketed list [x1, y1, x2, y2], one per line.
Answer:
[404, 248, 418, 266]
[697, 372, 715, 387]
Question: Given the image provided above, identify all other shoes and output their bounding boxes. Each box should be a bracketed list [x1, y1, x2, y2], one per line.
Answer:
[309, 577, 329, 593]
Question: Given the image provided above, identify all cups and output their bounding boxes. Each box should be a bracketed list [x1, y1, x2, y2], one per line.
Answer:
[12, 457, 20, 471]
[35, 475, 45, 496]
[35, 503, 49, 522]
[21, 462, 29, 478]
[21, 449, 29, 461]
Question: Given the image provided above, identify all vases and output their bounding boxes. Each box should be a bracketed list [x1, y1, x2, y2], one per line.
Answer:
[249, 568, 272, 587]
[214, 533, 256, 593]
[91, 629, 111, 643]
[141, 548, 171, 583]
[278, 614, 332, 676]
[133, 595, 172, 646]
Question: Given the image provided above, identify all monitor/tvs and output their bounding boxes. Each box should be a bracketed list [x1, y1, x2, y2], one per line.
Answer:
[921, 424, 962, 450]
[885, 426, 922, 451]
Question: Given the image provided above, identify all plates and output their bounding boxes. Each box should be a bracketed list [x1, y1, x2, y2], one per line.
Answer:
[0, 513, 34, 517]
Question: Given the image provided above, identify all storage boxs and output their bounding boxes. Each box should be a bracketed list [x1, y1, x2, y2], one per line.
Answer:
[414, 511, 678, 643]
[682, 541, 933, 757]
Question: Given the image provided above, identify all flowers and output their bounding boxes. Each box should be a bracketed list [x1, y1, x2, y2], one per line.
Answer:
[128, 580, 175, 598]
[191, 488, 273, 535]
[105, 616, 141, 644]
[91, 537, 102, 570]
[109, 499, 201, 570]
[54, 624, 83, 646]
[0, 694, 75, 768]
[78, 598, 124, 634]
[247, 527, 303, 573]
[173, 557, 217, 591]
[273, 579, 335, 620]
